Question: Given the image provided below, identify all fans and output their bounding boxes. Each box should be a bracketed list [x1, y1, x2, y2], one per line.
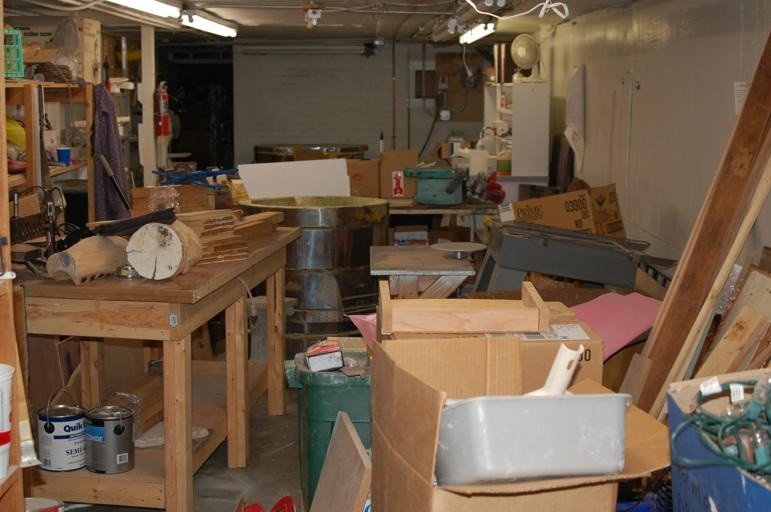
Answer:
[511, 35, 541, 81]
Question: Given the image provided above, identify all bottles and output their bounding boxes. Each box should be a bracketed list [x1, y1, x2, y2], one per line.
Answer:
[501, 93, 507, 108]
[101, 62, 106, 82]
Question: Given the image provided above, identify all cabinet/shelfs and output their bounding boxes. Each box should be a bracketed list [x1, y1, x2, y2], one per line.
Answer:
[1, 76, 135, 269]
[454, 80, 547, 177]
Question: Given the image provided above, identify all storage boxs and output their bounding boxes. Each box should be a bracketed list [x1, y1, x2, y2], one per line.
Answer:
[666, 368, 770, 512]
[380, 150, 417, 200]
[370, 338, 671, 512]
[346, 158, 381, 198]
[499, 183, 626, 239]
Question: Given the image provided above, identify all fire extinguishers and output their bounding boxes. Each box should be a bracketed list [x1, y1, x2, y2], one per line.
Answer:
[153, 81, 171, 136]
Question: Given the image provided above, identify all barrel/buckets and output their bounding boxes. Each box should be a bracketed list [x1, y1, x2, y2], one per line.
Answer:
[37, 388, 85, 472]
[85, 394, 134, 473]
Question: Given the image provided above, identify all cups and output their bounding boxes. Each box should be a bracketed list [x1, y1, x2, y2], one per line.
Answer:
[56, 148, 72, 167]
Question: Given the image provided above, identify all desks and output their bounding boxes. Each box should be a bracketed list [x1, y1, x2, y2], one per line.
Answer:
[13, 210, 302, 512]
[370, 245, 477, 298]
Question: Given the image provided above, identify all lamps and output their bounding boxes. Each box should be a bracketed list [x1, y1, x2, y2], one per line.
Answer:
[107, 0, 182, 19]
[460, 23, 495, 46]
[179, 11, 239, 40]
[242, 45, 366, 56]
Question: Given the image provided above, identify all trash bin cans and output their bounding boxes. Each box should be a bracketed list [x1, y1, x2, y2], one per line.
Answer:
[282, 351, 373, 512]
